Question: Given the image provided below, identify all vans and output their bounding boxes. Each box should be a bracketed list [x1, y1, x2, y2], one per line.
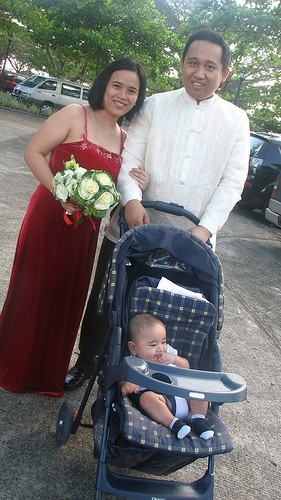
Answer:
[11, 74, 92, 118]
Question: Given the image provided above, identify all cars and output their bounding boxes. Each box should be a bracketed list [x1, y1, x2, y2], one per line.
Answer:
[0, 69, 31, 93]
[237, 131, 281, 219]
[264, 172, 281, 227]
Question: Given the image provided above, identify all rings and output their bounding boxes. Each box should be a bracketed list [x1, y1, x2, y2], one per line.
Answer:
[140, 179, 142, 183]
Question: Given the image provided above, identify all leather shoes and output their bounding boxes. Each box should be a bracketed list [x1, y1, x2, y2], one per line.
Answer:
[64, 365, 90, 391]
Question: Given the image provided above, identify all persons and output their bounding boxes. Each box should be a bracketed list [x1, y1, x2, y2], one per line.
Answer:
[0, 56, 151, 395]
[59, 28, 251, 392]
[120, 313, 215, 441]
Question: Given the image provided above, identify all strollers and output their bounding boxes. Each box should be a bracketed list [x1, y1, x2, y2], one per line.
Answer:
[56, 197, 247, 499]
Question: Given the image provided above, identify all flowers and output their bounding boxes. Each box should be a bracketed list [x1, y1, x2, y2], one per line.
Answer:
[52, 153, 121, 224]
